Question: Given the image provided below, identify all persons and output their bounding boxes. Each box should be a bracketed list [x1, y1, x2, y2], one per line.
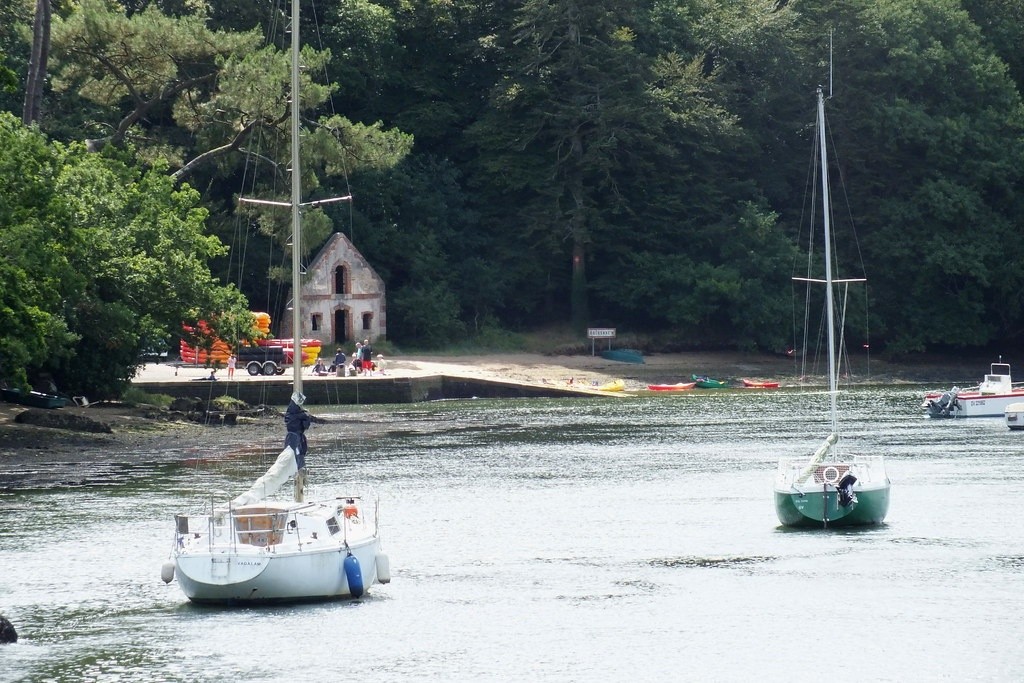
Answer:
[377, 354, 387, 376]
[227, 354, 237, 380]
[361, 339, 373, 376]
[313, 353, 361, 374]
[344, 499, 360, 519]
[334, 348, 346, 365]
[356, 343, 363, 360]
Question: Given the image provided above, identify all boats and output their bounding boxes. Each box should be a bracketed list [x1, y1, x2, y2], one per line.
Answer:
[920, 353, 1024, 430]
[593, 379, 625, 391]
[648, 382, 697, 390]
[743, 378, 778, 388]
[692, 373, 729, 389]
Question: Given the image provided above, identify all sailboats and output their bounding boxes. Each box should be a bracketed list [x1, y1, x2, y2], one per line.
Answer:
[170, 1, 383, 606]
[773, 82, 892, 531]
